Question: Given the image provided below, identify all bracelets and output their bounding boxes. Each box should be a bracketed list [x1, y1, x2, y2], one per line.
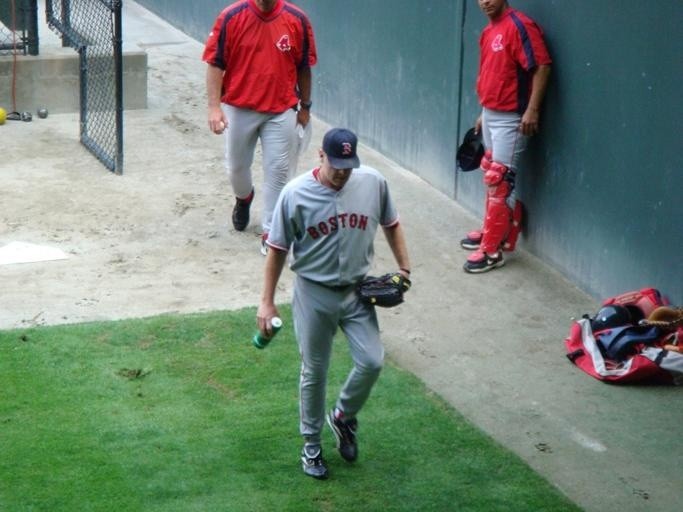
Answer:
[401, 267, 411, 275]
[298, 99, 311, 113]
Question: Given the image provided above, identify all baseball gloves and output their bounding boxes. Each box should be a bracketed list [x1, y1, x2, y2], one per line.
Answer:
[356, 270, 411, 307]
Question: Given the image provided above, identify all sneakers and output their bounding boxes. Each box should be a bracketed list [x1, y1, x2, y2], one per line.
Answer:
[301, 442, 328, 479]
[461, 236, 481, 250]
[463, 247, 504, 273]
[232, 187, 254, 230]
[326, 408, 358, 461]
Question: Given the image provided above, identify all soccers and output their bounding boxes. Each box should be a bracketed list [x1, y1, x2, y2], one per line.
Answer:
[0, 109, 6, 125]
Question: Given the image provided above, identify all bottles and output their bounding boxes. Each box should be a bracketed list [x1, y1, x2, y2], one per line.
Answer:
[253, 316, 283, 349]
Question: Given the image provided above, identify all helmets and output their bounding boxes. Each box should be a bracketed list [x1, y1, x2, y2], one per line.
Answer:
[590, 302, 631, 330]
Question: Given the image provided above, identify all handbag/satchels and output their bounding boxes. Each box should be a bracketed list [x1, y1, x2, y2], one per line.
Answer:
[565, 284, 683, 387]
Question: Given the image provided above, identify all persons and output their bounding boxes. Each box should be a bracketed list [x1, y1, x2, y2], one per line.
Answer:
[199, 1, 316, 255]
[459, 0, 554, 274]
[254, 128, 413, 479]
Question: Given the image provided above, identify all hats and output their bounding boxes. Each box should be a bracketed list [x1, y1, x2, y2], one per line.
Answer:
[456, 127, 484, 171]
[323, 127, 359, 169]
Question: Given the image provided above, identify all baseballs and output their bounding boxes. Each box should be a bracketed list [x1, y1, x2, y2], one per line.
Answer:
[219, 120, 227, 133]
[39, 109, 48, 118]
[22, 112, 32, 121]
